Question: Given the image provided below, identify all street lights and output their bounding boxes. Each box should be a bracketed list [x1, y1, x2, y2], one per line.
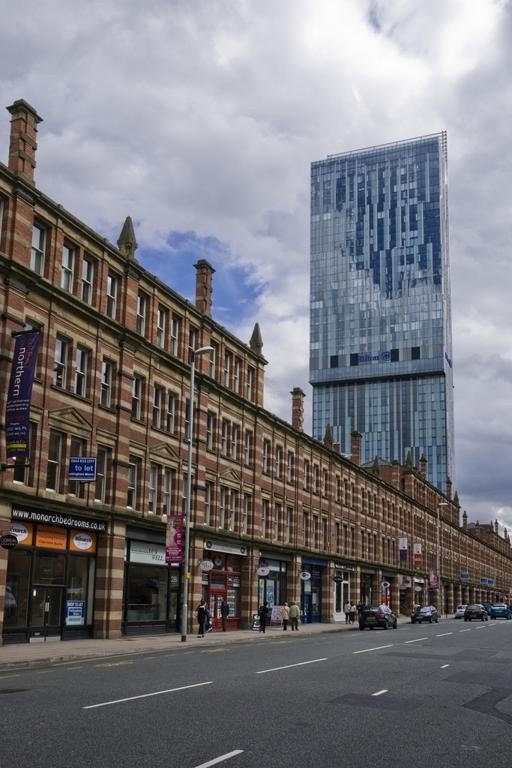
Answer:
[180, 343, 216, 647]
[435, 501, 451, 617]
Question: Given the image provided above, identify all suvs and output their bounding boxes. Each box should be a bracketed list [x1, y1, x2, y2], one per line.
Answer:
[455, 602, 512, 624]
[359, 604, 399, 631]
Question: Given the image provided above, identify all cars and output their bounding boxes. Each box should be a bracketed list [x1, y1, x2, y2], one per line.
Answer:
[1, 583, 20, 626]
[411, 605, 440, 625]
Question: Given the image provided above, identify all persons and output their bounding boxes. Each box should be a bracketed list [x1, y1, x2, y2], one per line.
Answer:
[343, 600, 350, 623]
[197, 598, 212, 638]
[280, 600, 291, 631]
[350, 601, 356, 625]
[258, 602, 270, 634]
[220, 596, 231, 632]
[289, 601, 301, 630]
